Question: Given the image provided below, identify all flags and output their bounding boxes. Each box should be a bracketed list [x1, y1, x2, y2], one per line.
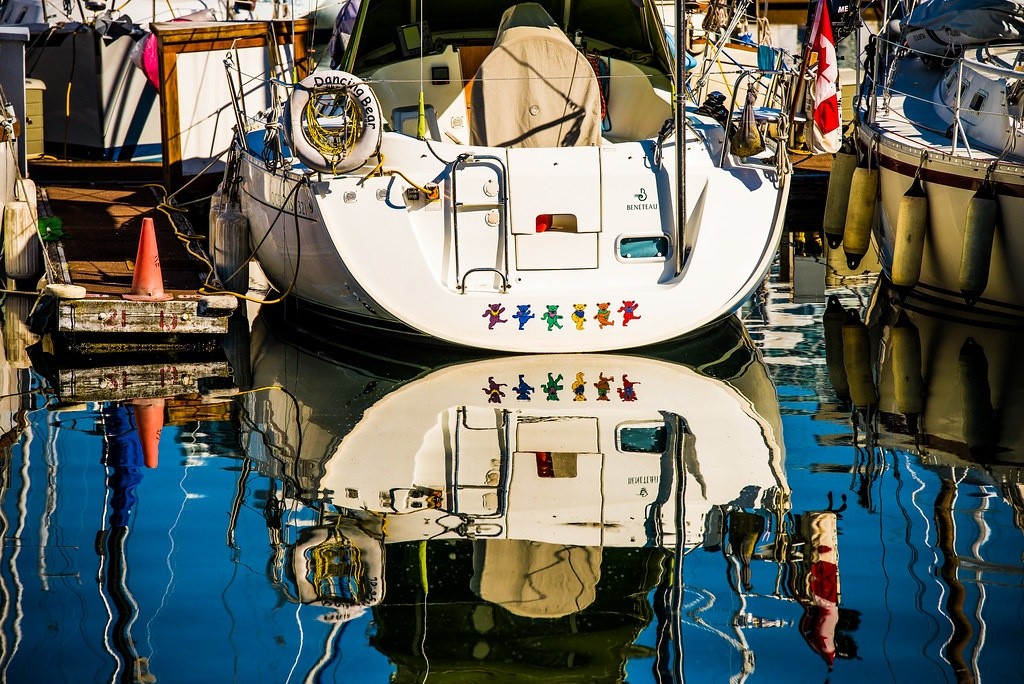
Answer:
[806, 0, 843, 157]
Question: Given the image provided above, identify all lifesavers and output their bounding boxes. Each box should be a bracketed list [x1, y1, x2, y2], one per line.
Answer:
[288, 522, 387, 626]
[284, 69, 384, 174]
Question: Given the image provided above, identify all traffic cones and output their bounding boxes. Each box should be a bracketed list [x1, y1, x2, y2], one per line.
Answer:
[124, 396, 173, 468]
[120, 217, 174, 302]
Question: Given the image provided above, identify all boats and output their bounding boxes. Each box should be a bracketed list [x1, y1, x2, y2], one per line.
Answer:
[1, 0, 1024, 332]
[214, 0, 827, 356]
[850, 272, 1024, 684]
[239, 290, 805, 684]
